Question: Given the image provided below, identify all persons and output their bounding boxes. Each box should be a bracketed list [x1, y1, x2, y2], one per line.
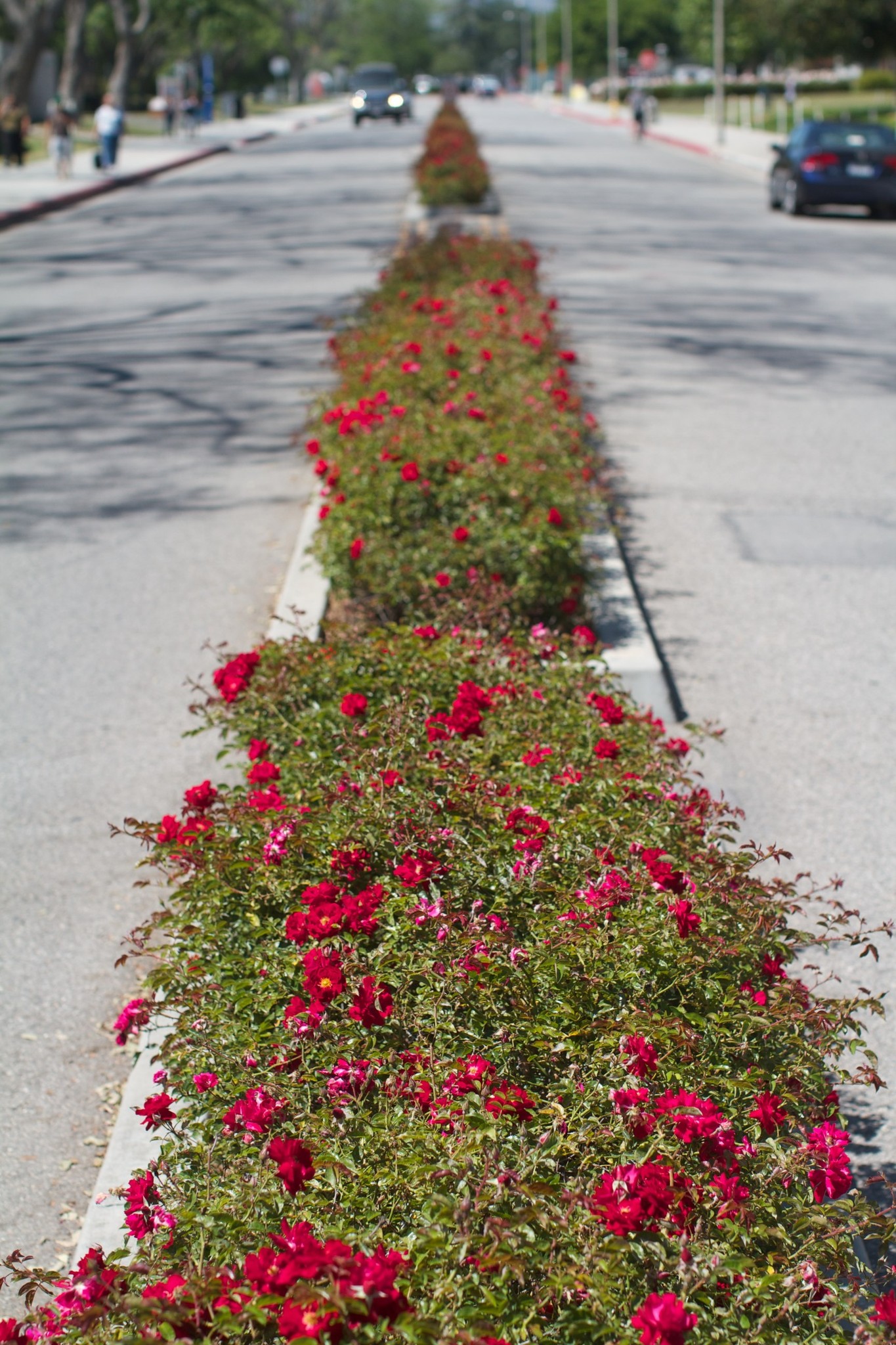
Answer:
[46, 97, 78, 177]
[93, 94, 122, 168]
[0, 91, 31, 167]
[163, 90, 200, 141]
[624, 87, 646, 141]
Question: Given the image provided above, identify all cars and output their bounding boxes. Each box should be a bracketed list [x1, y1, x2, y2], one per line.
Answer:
[350, 63, 500, 128]
[766, 120, 895, 221]
